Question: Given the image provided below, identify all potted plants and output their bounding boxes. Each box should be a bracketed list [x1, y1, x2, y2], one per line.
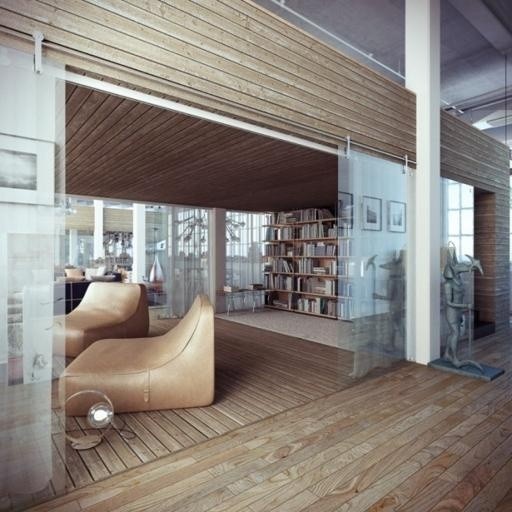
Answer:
[6, 323, 38, 386]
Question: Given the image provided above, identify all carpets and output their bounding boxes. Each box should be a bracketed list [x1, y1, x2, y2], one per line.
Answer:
[214, 307, 403, 357]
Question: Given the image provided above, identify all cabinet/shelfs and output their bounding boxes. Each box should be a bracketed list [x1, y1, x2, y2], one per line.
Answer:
[262, 216, 360, 321]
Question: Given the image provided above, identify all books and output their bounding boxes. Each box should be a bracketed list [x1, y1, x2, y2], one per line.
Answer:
[257, 208, 356, 323]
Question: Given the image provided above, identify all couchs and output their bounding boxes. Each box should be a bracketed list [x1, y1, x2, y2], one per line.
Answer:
[53, 271, 121, 315]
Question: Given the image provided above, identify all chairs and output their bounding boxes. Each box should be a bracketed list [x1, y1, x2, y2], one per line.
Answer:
[32, 281, 150, 358]
[57, 293, 215, 417]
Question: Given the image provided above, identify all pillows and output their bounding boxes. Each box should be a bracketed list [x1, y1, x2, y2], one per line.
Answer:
[64, 268, 82, 277]
[85, 268, 97, 279]
[66, 276, 86, 282]
[89, 275, 115, 282]
[97, 264, 108, 276]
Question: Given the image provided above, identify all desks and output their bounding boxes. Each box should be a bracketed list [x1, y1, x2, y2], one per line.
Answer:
[222, 288, 271, 315]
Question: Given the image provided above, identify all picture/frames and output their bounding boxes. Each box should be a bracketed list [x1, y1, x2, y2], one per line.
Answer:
[0, 132, 57, 206]
[387, 200, 407, 233]
[337, 191, 354, 229]
[361, 195, 383, 231]
